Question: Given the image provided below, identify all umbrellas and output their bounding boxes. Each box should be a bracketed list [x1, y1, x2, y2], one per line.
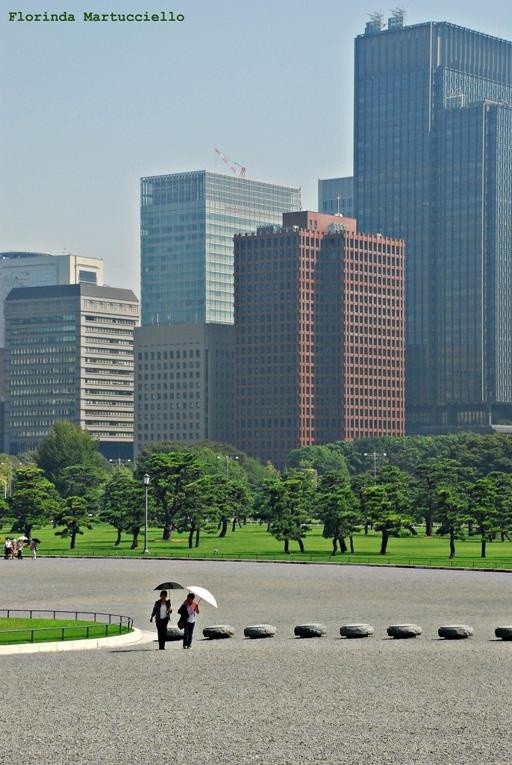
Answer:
[154, 582, 184, 611]
[186, 586, 217, 611]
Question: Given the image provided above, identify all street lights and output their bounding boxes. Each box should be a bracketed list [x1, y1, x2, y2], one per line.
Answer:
[142, 472, 152, 554]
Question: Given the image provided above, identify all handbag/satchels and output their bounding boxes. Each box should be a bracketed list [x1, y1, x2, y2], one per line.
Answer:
[178, 618, 184, 629]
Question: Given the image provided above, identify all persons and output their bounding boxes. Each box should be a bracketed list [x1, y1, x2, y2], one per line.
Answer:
[150, 591, 172, 650]
[178, 593, 199, 649]
[3, 535, 40, 560]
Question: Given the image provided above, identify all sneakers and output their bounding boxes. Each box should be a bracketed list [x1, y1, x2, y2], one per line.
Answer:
[184, 646, 192, 649]
